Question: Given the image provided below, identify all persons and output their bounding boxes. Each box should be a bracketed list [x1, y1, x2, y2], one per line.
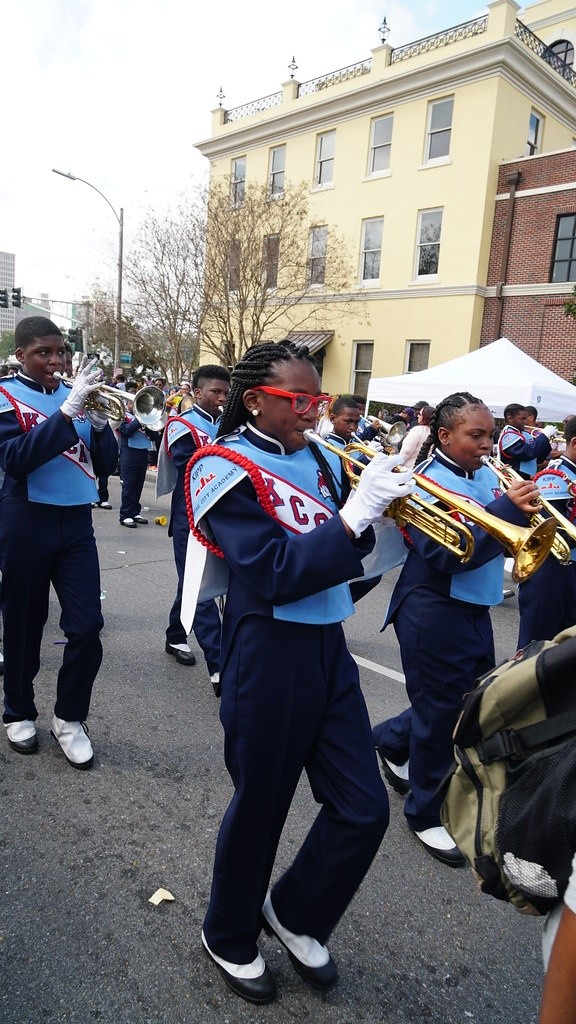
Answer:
[317, 394, 434, 469]
[178, 341, 414, 1006]
[372, 390, 542, 866]
[156, 365, 230, 666]
[496, 403, 576, 652]
[0, 316, 118, 769]
[540, 853, 576, 1024]
[65, 344, 189, 528]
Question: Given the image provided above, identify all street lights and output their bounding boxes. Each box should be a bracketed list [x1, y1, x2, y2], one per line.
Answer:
[52, 166, 125, 383]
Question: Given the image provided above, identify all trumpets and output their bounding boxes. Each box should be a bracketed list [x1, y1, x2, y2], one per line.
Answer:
[523, 425, 567, 444]
[146, 411, 168, 432]
[563, 474, 576, 498]
[218, 405, 224, 413]
[479, 455, 576, 564]
[302, 428, 557, 584]
[359, 414, 407, 447]
[350, 432, 384, 462]
[53, 371, 166, 424]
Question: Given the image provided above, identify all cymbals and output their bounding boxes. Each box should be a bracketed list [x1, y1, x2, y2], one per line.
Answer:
[177, 396, 196, 414]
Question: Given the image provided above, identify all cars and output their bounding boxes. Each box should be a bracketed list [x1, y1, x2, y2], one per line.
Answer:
[119, 354, 133, 364]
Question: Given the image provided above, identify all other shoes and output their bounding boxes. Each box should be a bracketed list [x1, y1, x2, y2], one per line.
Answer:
[3, 719, 37, 754]
[261, 892, 337, 990]
[413, 826, 465, 866]
[147, 465, 157, 472]
[100, 501, 112, 509]
[134, 515, 148, 524]
[121, 518, 137, 528]
[376, 748, 410, 795]
[49, 714, 94, 769]
[201, 930, 277, 1006]
[163, 641, 196, 666]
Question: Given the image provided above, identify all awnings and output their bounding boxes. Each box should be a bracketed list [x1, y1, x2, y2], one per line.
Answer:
[284, 332, 333, 354]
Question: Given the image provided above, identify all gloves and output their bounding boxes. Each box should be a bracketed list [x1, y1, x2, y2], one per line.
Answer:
[60, 354, 106, 418]
[339, 451, 415, 538]
[86, 396, 109, 432]
[542, 425, 558, 438]
[366, 441, 384, 461]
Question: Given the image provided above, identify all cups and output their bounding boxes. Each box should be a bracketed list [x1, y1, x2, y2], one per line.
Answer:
[155, 516, 167, 525]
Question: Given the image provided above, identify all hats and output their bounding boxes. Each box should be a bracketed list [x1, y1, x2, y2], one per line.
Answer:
[410, 401, 428, 409]
[405, 408, 414, 417]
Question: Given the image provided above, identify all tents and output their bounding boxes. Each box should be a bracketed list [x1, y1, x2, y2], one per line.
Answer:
[364, 338, 576, 417]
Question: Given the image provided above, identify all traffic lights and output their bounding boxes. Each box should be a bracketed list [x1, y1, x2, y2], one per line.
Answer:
[68, 328, 84, 352]
[0, 288, 10, 308]
[12, 287, 22, 309]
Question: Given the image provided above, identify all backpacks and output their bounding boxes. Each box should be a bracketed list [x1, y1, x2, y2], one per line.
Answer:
[437, 621, 576, 916]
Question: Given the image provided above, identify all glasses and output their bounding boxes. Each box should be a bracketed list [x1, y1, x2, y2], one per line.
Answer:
[403, 411, 407, 414]
[155, 383, 163, 386]
[250, 385, 334, 415]
